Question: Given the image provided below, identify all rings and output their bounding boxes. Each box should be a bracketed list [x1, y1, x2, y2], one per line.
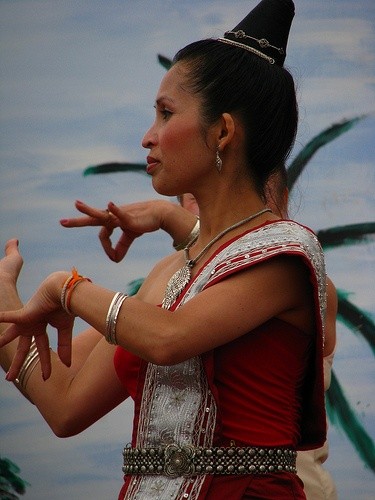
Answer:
[104, 209, 112, 227]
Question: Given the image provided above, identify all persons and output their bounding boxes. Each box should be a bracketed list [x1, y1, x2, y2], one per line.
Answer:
[58, 164, 339, 500]
[0, 0, 340, 500]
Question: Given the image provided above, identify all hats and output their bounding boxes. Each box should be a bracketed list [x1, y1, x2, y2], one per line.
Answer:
[210, 0, 295, 68]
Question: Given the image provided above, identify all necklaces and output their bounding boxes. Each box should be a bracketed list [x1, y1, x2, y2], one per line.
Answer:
[183, 208, 272, 268]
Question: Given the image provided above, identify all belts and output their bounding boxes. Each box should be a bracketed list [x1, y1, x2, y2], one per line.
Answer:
[122, 444, 297, 479]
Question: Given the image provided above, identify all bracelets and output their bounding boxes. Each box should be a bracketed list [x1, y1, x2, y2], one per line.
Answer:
[59, 266, 129, 344]
[15, 337, 52, 391]
[173, 215, 200, 251]
